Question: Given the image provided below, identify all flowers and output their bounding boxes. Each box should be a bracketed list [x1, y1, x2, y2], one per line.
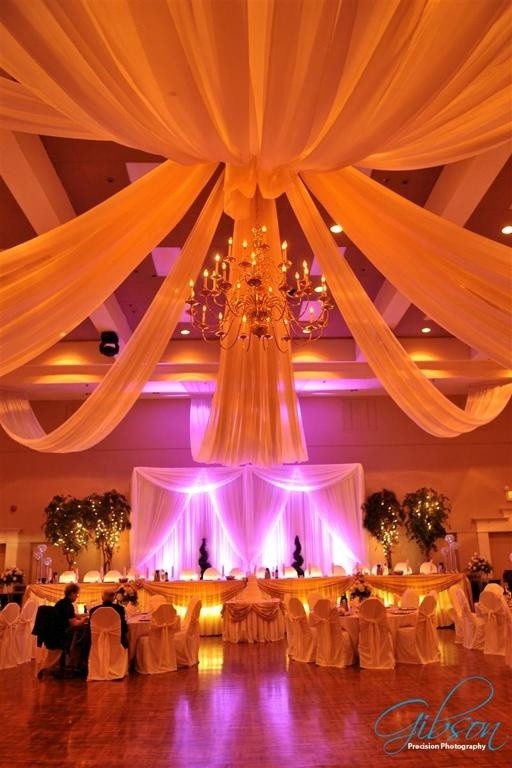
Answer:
[0, 566, 25, 587]
[465, 552, 496, 578]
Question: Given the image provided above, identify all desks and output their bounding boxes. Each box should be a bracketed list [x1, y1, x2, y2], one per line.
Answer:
[223, 602, 284, 643]
[21, 575, 474, 637]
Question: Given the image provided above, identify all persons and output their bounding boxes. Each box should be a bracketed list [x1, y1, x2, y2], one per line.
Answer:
[53, 583, 84, 643]
[87, 590, 128, 648]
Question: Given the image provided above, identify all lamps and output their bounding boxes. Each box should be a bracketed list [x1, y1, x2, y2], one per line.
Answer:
[175, 198, 334, 354]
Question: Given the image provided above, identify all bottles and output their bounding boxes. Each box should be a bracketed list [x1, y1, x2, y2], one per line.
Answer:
[265, 566, 279, 578]
[340, 593, 348, 612]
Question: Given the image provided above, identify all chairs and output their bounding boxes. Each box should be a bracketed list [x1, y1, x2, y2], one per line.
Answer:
[449, 583, 512, 656]
[284, 590, 439, 670]
[0, 595, 202, 681]
